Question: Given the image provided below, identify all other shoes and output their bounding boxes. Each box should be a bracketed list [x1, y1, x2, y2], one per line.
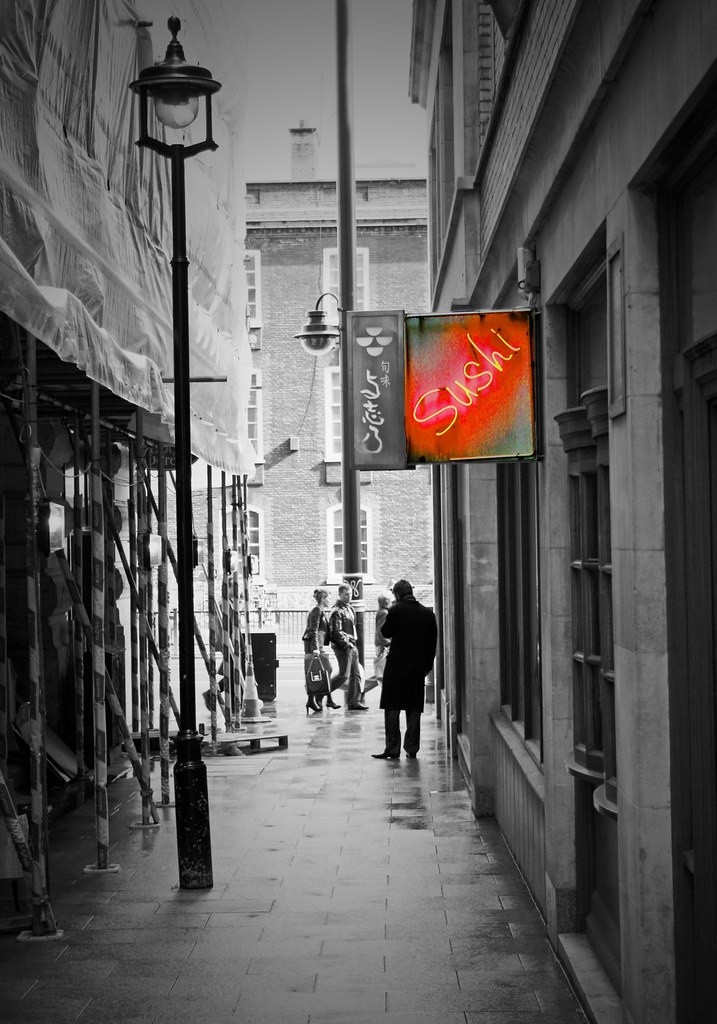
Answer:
[348, 702, 369, 710]
[315, 697, 323, 708]
[406, 753, 416, 759]
[371, 752, 400, 759]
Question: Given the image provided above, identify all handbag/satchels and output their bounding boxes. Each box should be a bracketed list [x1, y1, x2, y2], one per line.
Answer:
[302, 629, 325, 653]
[306, 654, 330, 696]
[304, 653, 332, 673]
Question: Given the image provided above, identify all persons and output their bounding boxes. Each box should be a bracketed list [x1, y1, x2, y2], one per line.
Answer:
[371, 579, 437, 759]
[360, 594, 391, 701]
[302, 588, 342, 714]
[315, 583, 369, 710]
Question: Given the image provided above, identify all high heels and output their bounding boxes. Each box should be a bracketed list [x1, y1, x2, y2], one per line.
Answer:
[306, 702, 323, 714]
[326, 702, 342, 711]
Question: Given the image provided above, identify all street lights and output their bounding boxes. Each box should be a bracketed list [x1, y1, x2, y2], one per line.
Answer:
[294, 292, 343, 355]
[130, 16, 224, 889]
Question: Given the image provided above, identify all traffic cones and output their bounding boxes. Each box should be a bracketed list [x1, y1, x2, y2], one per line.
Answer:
[230, 653, 270, 724]
